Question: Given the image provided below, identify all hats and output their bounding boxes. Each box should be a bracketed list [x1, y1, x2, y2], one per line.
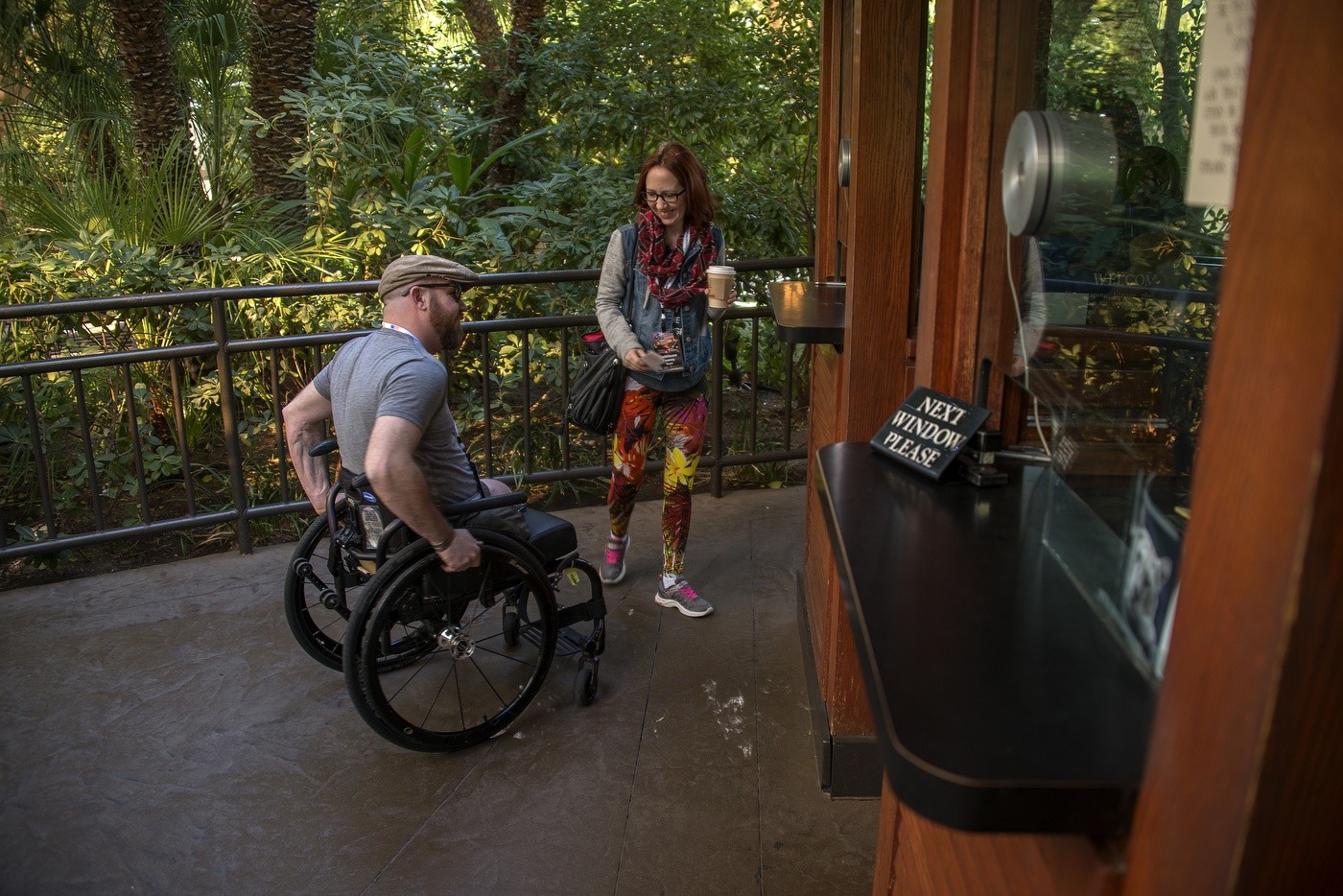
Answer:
[378, 254, 481, 301]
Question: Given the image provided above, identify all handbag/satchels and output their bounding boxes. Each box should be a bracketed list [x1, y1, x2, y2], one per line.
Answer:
[566, 347, 628, 433]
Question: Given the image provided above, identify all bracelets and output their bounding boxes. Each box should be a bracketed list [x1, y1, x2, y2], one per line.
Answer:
[431, 526, 455, 551]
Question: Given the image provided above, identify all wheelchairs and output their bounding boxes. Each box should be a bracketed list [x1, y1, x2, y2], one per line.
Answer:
[284, 430, 606, 755]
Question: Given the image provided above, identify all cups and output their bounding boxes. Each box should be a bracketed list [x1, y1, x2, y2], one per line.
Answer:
[706, 265, 737, 309]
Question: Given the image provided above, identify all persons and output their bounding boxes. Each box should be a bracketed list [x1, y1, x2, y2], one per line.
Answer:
[1008, 235, 1048, 374]
[281, 255, 511, 571]
[568, 141, 735, 616]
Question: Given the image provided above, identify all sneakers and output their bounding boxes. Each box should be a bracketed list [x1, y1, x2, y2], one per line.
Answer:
[599, 532, 630, 583]
[655, 574, 714, 616]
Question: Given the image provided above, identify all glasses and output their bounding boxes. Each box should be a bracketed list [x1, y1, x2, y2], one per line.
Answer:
[642, 189, 686, 203]
[400, 283, 462, 302]
